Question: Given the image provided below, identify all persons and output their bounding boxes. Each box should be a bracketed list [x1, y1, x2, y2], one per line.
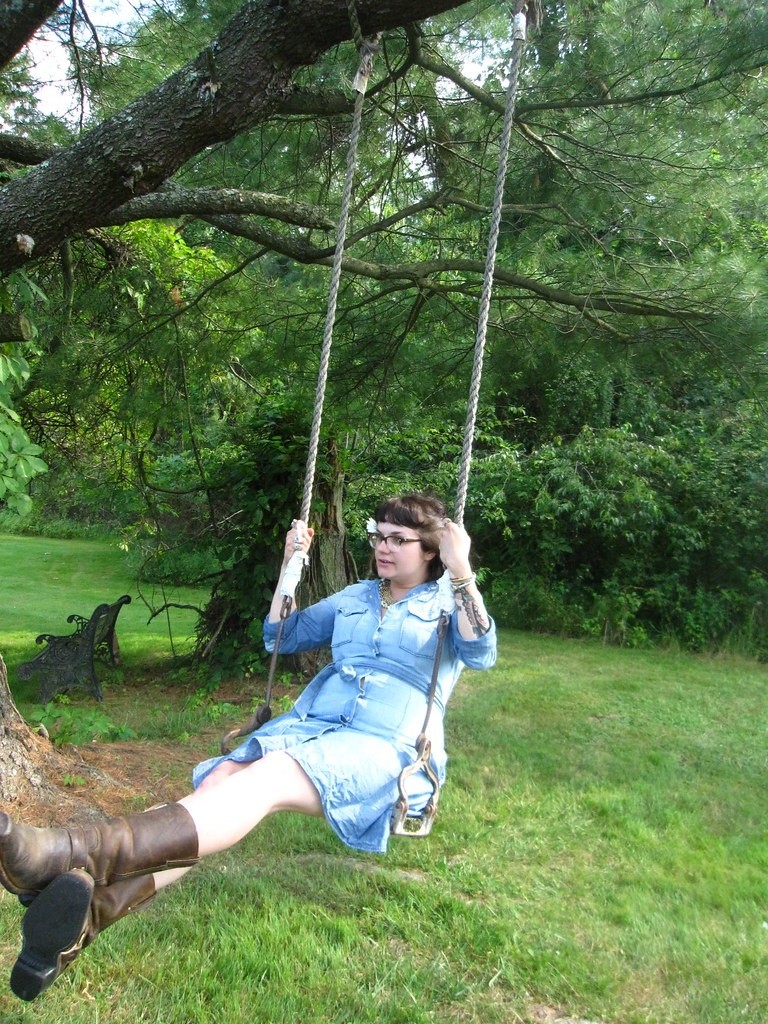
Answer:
[0, 494, 498, 1001]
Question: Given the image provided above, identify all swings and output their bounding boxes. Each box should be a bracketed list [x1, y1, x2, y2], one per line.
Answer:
[220, 0, 526, 835]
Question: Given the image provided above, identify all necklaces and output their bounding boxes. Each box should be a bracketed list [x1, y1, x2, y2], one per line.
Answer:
[378, 581, 398, 609]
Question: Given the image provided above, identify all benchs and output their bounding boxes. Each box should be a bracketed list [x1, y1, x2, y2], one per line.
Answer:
[13, 593, 132, 704]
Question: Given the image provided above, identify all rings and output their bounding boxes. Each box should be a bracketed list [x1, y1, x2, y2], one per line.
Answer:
[295, 544, 301, 550]
[294, 536, 299, 543]
[444, 519, 451, 527]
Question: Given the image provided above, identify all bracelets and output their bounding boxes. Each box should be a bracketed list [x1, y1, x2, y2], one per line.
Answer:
[450, 572, 476, 592]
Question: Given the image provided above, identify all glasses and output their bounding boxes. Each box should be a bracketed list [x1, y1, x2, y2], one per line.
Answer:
[366, 532, 422, 553]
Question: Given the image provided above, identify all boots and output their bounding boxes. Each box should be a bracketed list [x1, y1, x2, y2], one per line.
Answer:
[0, 803, 200, 907]
[10, 869, 157, 1001]
